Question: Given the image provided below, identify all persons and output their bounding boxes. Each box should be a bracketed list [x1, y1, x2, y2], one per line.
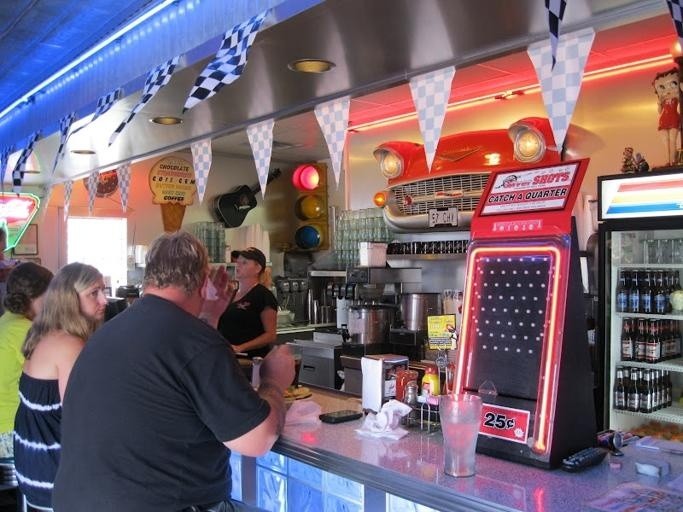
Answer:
[0, 228, 19, 314]
[0, 261, 53, 460]
[51, 228, 301, 512]
[13, 260, 107, 511]
[216, 246, 277, 360]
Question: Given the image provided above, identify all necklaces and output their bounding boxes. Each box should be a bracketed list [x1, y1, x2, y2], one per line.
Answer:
[234, 282, 258, 298]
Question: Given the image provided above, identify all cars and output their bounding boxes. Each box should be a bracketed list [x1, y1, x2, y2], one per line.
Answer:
[372, 116, 601, 233]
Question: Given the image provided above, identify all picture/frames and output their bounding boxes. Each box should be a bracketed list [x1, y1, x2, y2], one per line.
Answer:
[82, 170, 119, 198]
[10, 258, 42, 267]
[13, 223, 40, 256]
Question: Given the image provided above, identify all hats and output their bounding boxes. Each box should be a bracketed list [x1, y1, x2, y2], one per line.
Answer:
[231, 247, 265, 272]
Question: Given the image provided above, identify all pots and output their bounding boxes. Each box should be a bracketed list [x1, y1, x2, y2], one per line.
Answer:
[394, 290, 442, 334]
[346, 304, 396, 346]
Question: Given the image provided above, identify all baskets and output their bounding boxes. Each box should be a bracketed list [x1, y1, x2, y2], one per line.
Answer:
[389, 393, 442, 435]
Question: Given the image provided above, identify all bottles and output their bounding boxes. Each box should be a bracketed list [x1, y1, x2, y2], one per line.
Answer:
[251, 356, 261, 386]
[620, 316, 682, 364]
[613, 366, 675, 414]
[614, 265, 680, 315]
[402, 383, 417, 423]
[637, 237, 682, 264]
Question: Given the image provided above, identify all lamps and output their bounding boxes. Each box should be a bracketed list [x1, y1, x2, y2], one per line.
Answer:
[669, 35, 683, 64]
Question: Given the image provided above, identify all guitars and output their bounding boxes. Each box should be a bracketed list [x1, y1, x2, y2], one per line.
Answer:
[214, 167, 280, 227]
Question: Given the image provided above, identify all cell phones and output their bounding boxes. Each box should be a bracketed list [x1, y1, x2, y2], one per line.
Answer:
[319, 410, 363, 423]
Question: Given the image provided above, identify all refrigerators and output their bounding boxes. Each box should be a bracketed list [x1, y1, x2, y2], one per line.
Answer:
[595, 169, 683, 445]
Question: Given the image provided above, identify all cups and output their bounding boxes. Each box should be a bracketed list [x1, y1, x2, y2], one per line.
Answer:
[192, 220, 225, 261]
[334, 207, 391, 264]
[287, 345, 303, 388]
[387, 239, 468, 254]
[242, 225, 272, 264]
[438, 393, 483, 478]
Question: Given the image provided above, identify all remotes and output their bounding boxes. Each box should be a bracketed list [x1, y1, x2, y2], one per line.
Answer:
[560, 446, 608, 472]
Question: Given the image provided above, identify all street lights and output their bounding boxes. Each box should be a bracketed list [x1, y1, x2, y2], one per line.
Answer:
[671, 37, 682, 171]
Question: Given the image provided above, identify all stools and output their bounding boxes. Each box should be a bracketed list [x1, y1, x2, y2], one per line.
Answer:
[1, 457, 30, 512]
[20, 493, 54, 512]
